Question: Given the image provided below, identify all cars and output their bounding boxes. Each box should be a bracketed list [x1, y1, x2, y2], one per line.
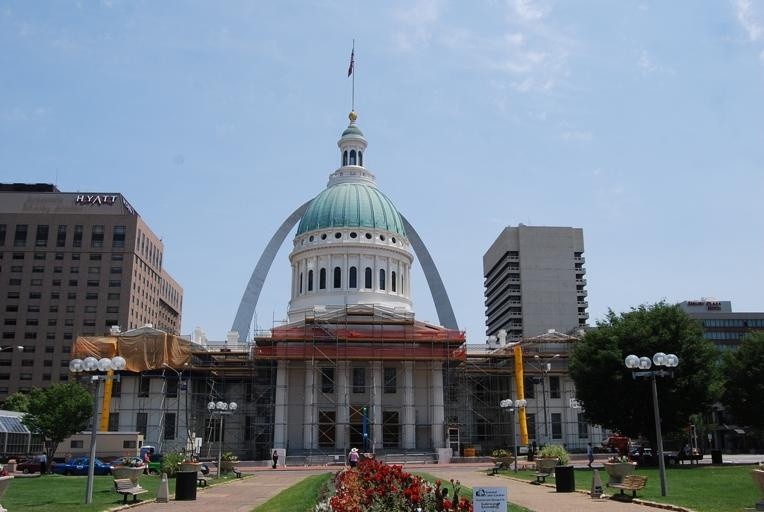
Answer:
[17, 458, 57, 475]
[110, 456, 143, 466]
[690, 448, 703, 459]
[47, 457, 113, 476]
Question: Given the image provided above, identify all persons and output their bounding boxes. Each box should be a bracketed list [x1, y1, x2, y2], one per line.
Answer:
[39, 451, 48, 475]
[586, 442, 594, 470]
[64, 451, 73, 462]
[272, 449, 279, 468]
[348, 447, 360, 468]
[143, 451, 151, 476]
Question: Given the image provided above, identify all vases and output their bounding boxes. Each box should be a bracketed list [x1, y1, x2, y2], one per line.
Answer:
[0, 476, 14, 512]
[110, 466, 145, 492]
[603, 462, 637, 486]
[178, 463, 201, 475]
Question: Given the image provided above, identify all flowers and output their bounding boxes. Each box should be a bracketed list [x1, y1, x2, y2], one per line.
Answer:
[115, 458, 144, 467]
[609, 455, 633, 463]
[180, 457, 199, 464]
[0, 467, 10, 477]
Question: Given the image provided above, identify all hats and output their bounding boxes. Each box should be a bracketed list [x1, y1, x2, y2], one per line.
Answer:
[351, 448, 358, 452]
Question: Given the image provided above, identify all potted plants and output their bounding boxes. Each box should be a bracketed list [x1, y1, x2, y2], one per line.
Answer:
[212, 452, 241, 475]
[533, 445, 559, 478]
[491, 449, 515, 471]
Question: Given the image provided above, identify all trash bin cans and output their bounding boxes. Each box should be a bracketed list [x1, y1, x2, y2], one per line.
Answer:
[175, 471, 197, 500]
[555, 465, 575, 492]
[711, 450, 722, 464]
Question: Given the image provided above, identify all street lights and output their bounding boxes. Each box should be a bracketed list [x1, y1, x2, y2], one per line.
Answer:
[206, 400, 238, 478]
[625, 351, 679, 497]
[0, 345, 25, 353]
[523, 354, 562, 437]
[499, 399, 528, 472]
[70, 355, 126, 504]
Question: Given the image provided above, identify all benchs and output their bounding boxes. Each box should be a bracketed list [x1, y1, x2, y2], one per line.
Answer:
[608, 475, 648, 498]
[675, 454, 704, 465]
[488, 462, 503, 475]
[530, 467, 552, 482]
[113, 479, 150, 502]
[197, 476, 207, 486]
[233, 466, 241, 477]
